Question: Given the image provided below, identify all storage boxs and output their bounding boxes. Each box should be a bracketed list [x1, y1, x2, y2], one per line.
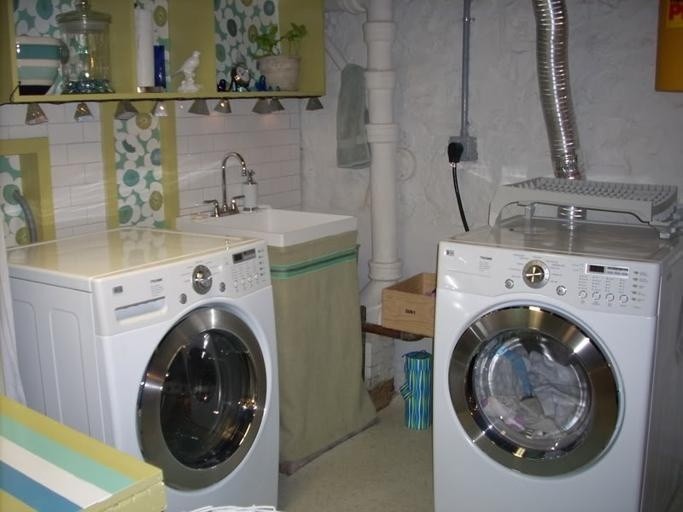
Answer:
[381, 272, 437, 338]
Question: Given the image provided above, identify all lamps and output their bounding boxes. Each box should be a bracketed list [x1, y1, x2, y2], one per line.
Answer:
[252, 97, 272, 114]
[73, 101, 94, 122]
[114, 100, 138, 120]
[306, 96, 323, 110]
[269, 98, 285, 111]
[214, 98, 233, 113]
[25, 102, 49, 125]
[152, 100, 168, 117]
[187, 97, 210, 114]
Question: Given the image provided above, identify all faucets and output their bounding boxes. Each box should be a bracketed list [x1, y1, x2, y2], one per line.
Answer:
[222, 153, 247, 202]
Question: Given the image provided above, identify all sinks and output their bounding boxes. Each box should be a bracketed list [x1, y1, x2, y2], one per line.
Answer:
[197, 209, 358, 247]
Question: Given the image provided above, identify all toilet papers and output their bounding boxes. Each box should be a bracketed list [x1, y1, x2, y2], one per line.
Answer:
[134, 10, 155, 88]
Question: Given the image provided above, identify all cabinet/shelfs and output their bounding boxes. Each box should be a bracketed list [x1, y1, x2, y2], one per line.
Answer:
[0, 0, 327, 109]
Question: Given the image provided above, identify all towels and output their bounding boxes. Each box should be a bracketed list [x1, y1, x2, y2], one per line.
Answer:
[335, 63, 373, 171]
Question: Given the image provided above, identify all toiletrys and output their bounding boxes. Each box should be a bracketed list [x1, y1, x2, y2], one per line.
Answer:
[244, 170, 258, 207]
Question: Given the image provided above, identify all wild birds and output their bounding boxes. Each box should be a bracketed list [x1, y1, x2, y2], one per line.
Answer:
[175, 49, 201, 78]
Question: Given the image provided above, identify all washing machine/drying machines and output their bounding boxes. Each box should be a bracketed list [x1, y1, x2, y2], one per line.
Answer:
[433, 215, 683, 512]
[6, 227, 280, 512]
